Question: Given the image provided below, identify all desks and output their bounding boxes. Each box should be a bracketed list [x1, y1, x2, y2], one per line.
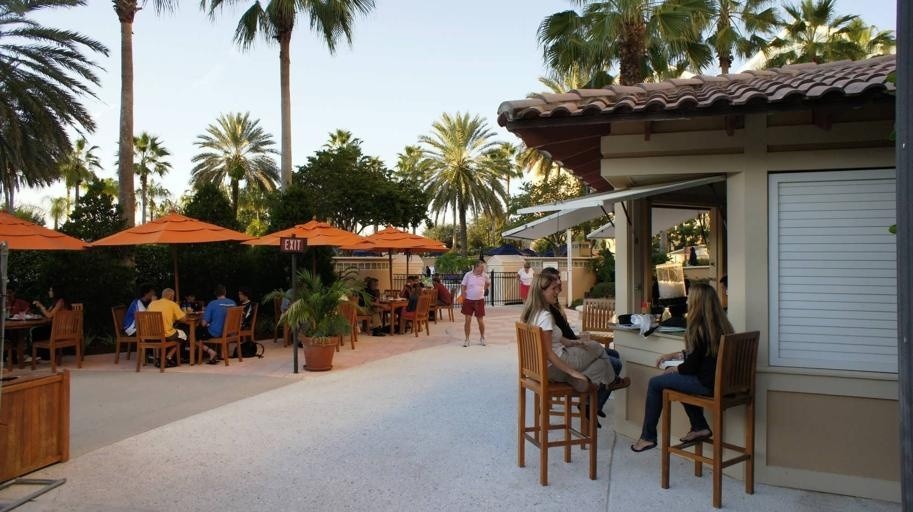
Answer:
[176, 313, 204, 366]
[2, 310, 49, 374]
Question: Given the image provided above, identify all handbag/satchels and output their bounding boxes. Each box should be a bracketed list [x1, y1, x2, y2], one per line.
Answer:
[233, 342, 256, 358]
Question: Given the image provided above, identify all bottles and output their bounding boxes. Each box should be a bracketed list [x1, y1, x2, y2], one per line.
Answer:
[379, 293, 401, 301]
[631, 299, 671, 324]
[181, 302, 201, 313]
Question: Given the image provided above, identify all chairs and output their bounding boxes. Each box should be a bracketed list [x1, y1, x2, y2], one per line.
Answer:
[658, 330, 760, 507]
[238, 301, 256, 343]
[133, 310, 182, 372]
[270, 276, 457, 354]
[67, 303, 84, 361]
[30, 308, 83, 372]
[110, 305, 148, 367]
[198, 305, 246, 367]
[512, 298, 619, 485]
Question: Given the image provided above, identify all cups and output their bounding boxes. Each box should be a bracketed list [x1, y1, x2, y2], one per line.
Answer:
[406, 283, 410, 286]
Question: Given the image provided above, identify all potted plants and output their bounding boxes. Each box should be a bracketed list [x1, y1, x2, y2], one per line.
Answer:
[257, 263, 376, 370]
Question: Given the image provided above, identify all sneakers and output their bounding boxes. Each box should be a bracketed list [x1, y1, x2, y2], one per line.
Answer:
[631, 440, 657, 452]
[609, 376, 630, 390]
[577, 403, 606, 428]
[679, 427, 712, 442]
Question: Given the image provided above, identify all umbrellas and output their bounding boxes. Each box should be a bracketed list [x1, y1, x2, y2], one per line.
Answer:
[0, 208, 91, 375]
[239, 220, 378, 336]
[82, 211, 260, 367]
[398, 246, 449, 279]
[336, 224, 450, 335]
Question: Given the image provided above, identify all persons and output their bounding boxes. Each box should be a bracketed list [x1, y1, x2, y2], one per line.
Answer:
[123, 282, 292, 365]
[356, 265, 447, 334]
[631, 284, 735, 452]
[720, 276, 728, 312]
[3, 281, 72, 365]
[515, 263, 534, 302]
[520, 267, 631, 429]
[460, 260, 490, 347]
